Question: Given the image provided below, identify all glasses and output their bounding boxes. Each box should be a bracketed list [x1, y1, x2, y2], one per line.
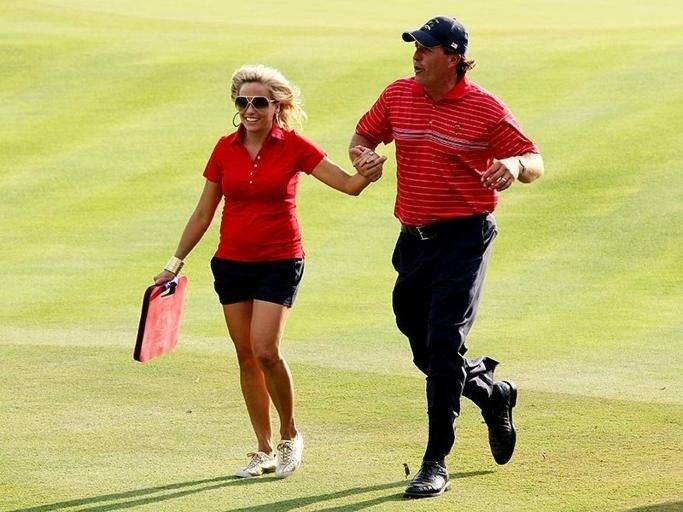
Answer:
[235, 95, 277, 111]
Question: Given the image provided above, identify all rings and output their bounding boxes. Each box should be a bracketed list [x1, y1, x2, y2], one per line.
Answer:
[502, 177, 507, 182]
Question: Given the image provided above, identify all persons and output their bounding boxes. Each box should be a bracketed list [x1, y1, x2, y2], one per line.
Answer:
[348, 17, 544, 497]
[153, 64, 382, 478]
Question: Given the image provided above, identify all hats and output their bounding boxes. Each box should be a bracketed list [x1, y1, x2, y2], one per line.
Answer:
[402, 15, 468, 54]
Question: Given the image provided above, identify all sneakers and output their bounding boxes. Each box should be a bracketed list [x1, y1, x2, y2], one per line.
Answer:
[235, 450, 277, 477]
[275, 430, 304, 479]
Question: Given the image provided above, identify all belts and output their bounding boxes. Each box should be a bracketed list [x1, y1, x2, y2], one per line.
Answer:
[400, 214, 483, 240]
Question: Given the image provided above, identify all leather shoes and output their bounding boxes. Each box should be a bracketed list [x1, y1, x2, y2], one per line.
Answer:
[403, 461, 451, 498]
[482, 380, 518, 465]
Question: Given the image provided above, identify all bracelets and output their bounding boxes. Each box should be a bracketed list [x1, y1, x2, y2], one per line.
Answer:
[164, 256, 184, 276]
[519, 158, 528, 174]
